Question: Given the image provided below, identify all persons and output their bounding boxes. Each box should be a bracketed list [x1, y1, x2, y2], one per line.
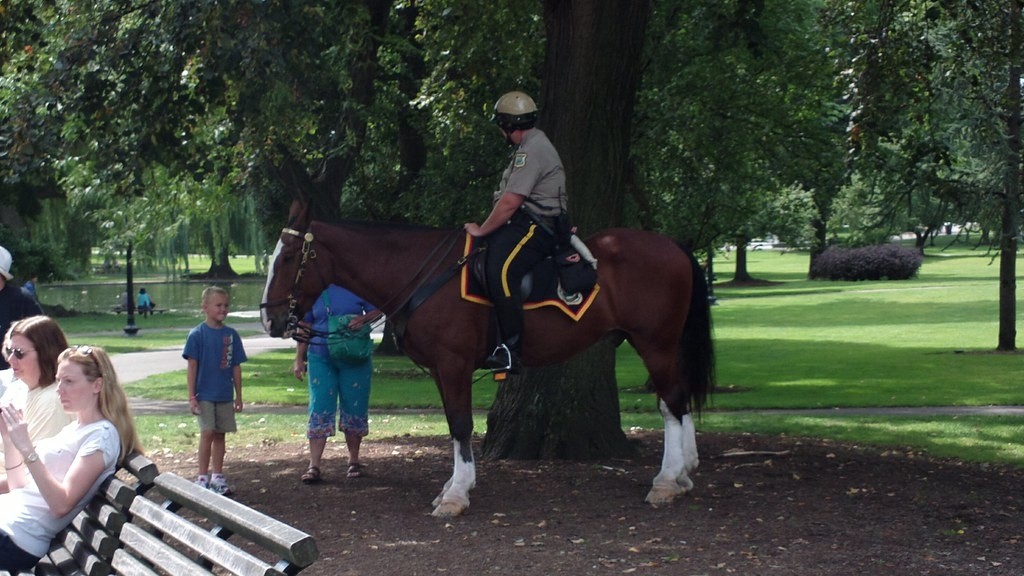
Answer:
[0, 315, 77, 494]
[138, 288, 154, 315]
[0, 345, 144, 570]
[294, 283, 382, 482]
[0, 245, 44, 370]
[182, 286, 248, 494]
[464, 91, 569, 374]
[25, 275, 38, 293]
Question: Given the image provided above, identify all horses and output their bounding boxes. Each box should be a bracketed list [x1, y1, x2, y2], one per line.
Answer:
[258, 195, 708, 517]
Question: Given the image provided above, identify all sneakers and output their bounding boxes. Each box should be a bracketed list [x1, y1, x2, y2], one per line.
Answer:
[209, 473, 232, 496]
[194, 475, 210, 489]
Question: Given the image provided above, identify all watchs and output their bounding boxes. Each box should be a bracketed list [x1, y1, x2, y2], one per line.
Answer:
[24, 453, 38, 463]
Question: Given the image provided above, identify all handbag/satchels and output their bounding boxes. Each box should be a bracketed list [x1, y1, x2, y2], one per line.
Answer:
[327, 314, 373, 363]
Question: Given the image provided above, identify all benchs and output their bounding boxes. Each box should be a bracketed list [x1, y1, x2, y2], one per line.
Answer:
[0, 449, 320, 576]
[111, 308, 169, 314]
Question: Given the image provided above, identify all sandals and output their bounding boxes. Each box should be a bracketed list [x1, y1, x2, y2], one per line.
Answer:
[346, 463, 361, 478]
[300, 466, 321, 482]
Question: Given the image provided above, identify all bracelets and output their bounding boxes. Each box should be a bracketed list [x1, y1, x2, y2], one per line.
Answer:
[190, 396, 195, 399]
[6, 462, 22, 470]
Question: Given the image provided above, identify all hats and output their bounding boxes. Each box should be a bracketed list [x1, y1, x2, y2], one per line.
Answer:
[0, 246, 14, 280]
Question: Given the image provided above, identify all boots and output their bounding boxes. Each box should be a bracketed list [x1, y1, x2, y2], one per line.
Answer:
[488, 305, 522, 375]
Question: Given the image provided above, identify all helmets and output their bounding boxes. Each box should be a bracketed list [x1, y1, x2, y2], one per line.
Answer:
[489, 91, 539, 130]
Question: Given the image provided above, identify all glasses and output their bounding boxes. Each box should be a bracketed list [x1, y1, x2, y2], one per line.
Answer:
[6, 347, 37, 359]
[68, 344, 102, 377]
